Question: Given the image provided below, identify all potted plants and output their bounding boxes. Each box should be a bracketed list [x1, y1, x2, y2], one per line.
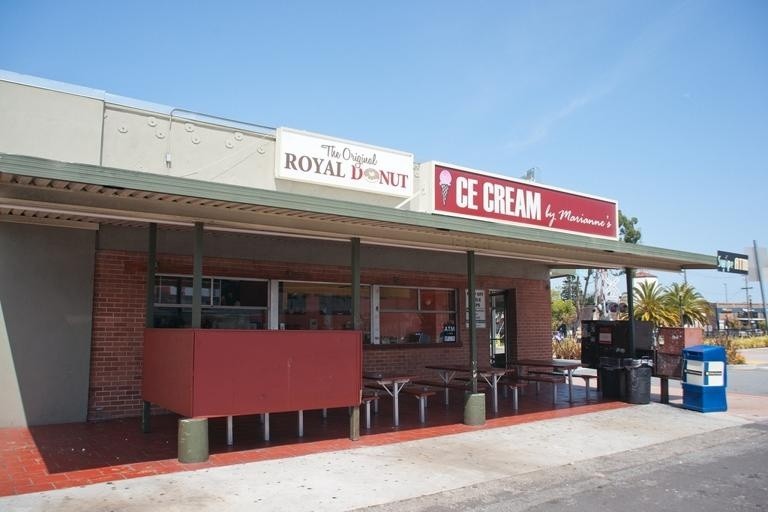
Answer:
[553, 336, 583, 368]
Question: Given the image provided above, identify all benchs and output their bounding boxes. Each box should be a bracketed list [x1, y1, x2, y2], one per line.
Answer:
[364, 385, 436, 424]
[501, 374, 565, 404]
[454, 377, 527, 410]
[323, 396, 380, 428]
[412, 380, 485, 407]
[529, 370, 598, 400]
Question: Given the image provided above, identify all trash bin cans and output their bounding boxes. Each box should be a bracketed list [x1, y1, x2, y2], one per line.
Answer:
[495, 352, 511, 383]
[597, 357, 652, 404]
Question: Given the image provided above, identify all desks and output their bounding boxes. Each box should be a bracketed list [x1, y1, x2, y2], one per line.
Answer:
[426, 363, 514, 414]
[362, 373, 420, 426]
[508, 358, 582, 404]
